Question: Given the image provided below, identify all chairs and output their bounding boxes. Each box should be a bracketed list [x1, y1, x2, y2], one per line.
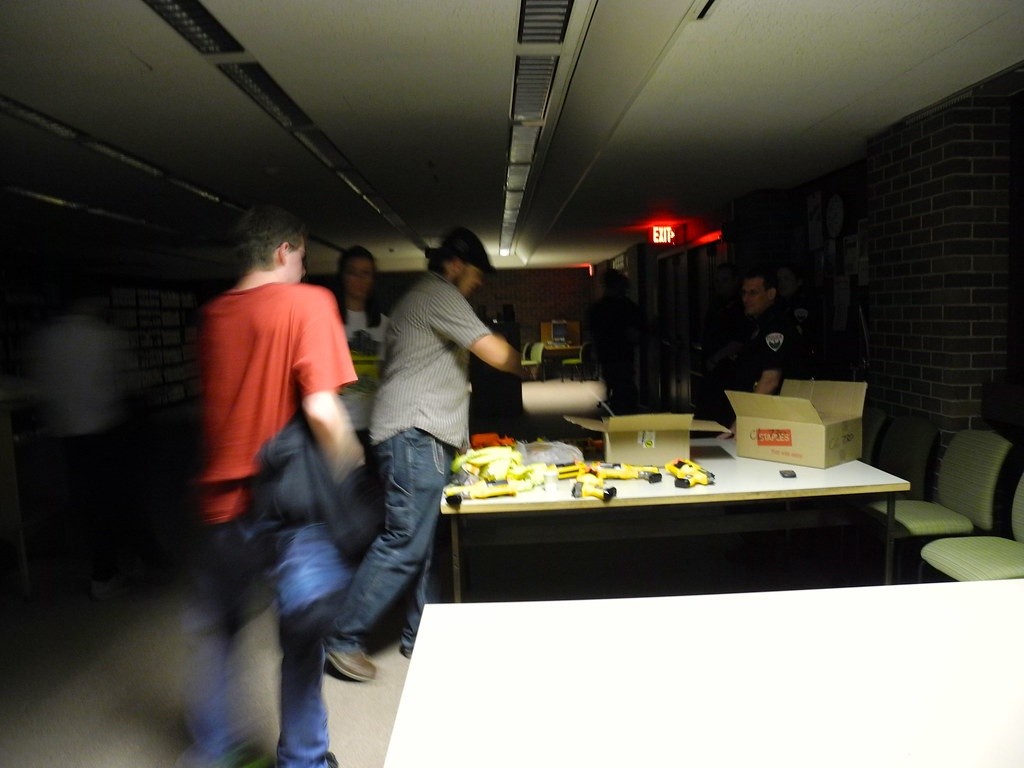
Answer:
[521, 342, 534, 381]
[918, 473, 1024, 583]
[561, 341, 593, 382]
[860, 430, 1017, 578]
[785, 406, 890, 546]
[521, 342, 546, 380]
[838, 416, 940, 559]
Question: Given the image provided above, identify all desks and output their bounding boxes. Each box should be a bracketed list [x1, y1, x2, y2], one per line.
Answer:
[542, 345, 581, 380]
[383, 578, 1024, 768]
[440, 437, 911, 603]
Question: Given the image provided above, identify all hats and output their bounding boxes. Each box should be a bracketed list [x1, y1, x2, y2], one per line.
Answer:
[443, 228, 498, 274]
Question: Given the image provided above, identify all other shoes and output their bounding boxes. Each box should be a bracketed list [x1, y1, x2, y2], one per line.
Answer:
[324, 642, 377, 682]
[400, 644, 414, 658]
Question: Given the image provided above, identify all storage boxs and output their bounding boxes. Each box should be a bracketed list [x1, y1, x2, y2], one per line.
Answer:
[724, 379, 867, 470]
[563, 413, 732, 467]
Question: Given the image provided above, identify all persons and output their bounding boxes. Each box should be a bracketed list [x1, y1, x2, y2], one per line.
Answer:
[324, 245, 391, 570]
[715, 270, 807, 440]
[174, 203, 366, 768]
[21, 275, 169, 597]
[325, 228, 536, 682]
[582, 267, 649, 407]
